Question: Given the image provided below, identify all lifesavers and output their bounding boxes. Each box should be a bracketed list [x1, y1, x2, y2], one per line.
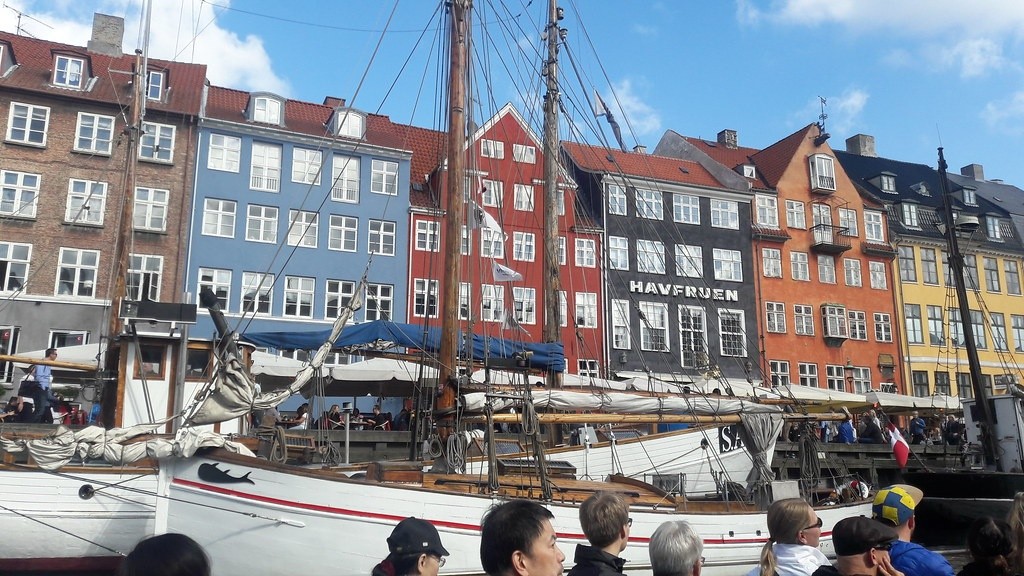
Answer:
[828, 481, 869, 505]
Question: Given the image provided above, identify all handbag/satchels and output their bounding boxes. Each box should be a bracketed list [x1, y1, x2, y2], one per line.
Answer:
[18, 381, 41, 398]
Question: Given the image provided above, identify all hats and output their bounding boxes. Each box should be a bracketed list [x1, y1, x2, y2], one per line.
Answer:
[387, 516, 450, 556]
[832, 515, 899, 555]
[872, 484, 923, 526]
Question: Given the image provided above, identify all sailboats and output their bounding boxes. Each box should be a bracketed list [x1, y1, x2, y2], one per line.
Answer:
[0, 0, 846, 572]
[0, 1, 876, 575]
[875, 147, 1024, 544]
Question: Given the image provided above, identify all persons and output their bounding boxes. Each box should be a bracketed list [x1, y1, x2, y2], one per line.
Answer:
[287, 403, 412, 431]
[747, 483, 1024, 576]
[371, 516, 450, 576]
[120, 532, 213, 576]
[0, 347, 86, 424]
[649, 520, 705, 576]
[566, 490, 632, 576]
[814, 409, 966, 444]
[536, 381, 542, 388]
[480, 499, 565, 576]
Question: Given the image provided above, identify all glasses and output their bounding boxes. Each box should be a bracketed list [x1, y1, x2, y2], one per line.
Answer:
[417, 551, 446, 567]
[796, 517, 822, 537]
[873, 544, 893, 556]
[693, 557, 705, 568]
[622, 519, 633, 529]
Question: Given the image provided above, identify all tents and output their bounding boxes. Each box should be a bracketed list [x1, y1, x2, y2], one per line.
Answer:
[317, 357, 442, 430]
[248, 350, 331, 401]
[463, 370, 963, 417]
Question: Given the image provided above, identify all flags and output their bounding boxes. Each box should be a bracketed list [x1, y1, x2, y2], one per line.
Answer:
[888, 420, 908, 467]
